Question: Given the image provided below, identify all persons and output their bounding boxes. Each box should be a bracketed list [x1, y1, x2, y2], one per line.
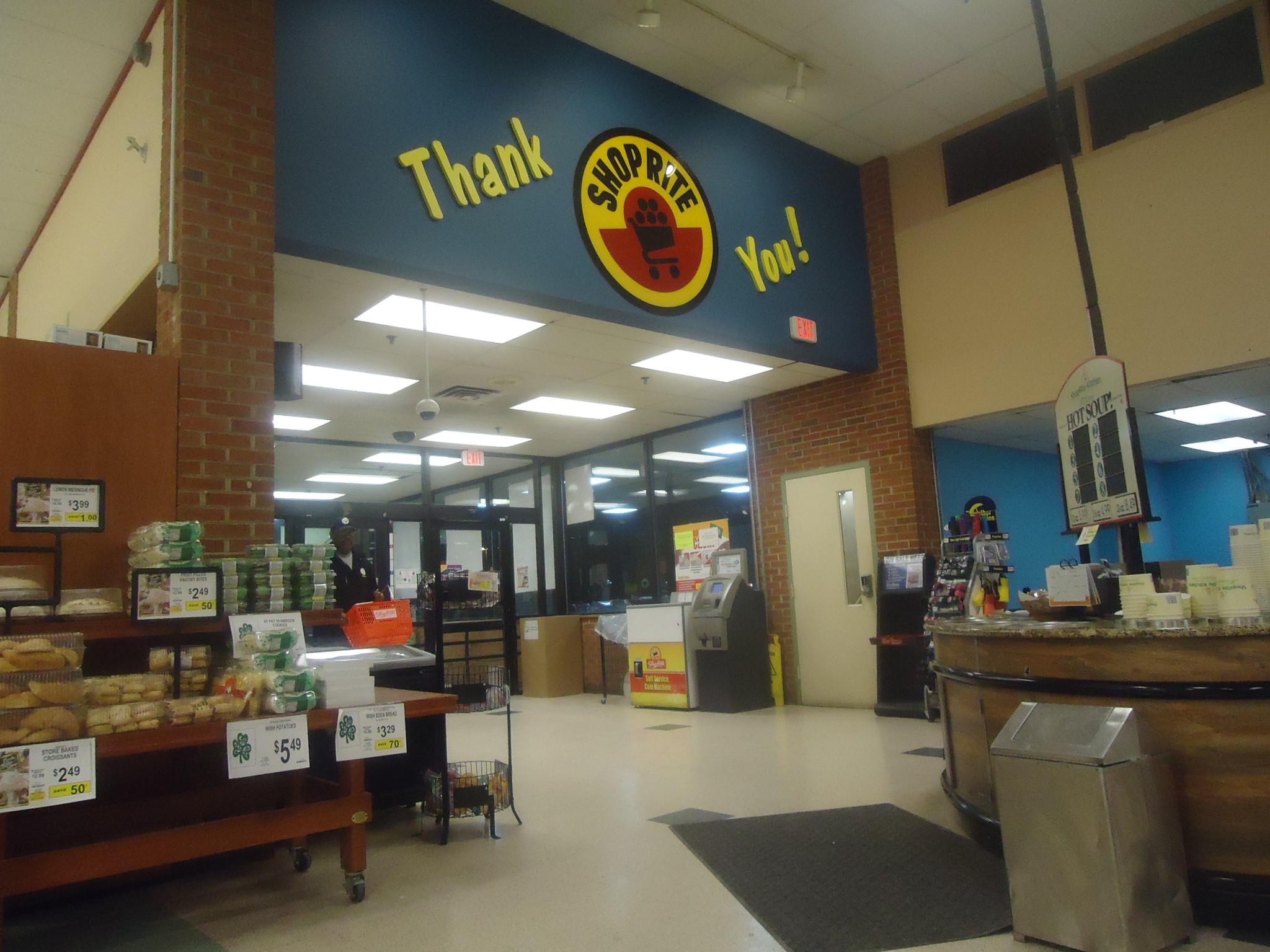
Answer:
[329, 520, 386, 626]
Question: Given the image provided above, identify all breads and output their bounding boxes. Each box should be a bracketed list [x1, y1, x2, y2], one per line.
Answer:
[0, 638, 261, 747]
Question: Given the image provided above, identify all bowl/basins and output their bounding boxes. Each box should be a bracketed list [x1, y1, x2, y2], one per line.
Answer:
[1085, 577, 1120, 614]
[1019, 599, 1076, 622]
[1118, 518, 1269, 622]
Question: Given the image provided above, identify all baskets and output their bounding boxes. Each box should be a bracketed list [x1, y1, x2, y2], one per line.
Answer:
[339, 586, 414, 647]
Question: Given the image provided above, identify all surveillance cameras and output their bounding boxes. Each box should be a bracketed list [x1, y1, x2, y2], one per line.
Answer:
[392, 431, 416, 443]
[415, 400, 441, 420]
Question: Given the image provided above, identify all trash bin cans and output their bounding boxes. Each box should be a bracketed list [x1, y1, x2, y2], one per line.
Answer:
[990, 701, 1194, 952]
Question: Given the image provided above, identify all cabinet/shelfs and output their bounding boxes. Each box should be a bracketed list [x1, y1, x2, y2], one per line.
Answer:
[0, 611, 458, 904]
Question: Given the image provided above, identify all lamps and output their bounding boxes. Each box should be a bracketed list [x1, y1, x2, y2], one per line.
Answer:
[787, 59, 805, 102]
[638, 0, 661, 28]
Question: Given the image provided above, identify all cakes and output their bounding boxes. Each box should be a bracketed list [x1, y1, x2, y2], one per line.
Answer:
[0, 576, 118, 619]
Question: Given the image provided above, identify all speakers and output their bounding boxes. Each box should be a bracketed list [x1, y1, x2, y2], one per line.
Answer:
[274, 342, 302, 402]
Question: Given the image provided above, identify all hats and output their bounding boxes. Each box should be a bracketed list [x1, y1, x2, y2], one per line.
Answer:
[330, 517, 355, 532]
